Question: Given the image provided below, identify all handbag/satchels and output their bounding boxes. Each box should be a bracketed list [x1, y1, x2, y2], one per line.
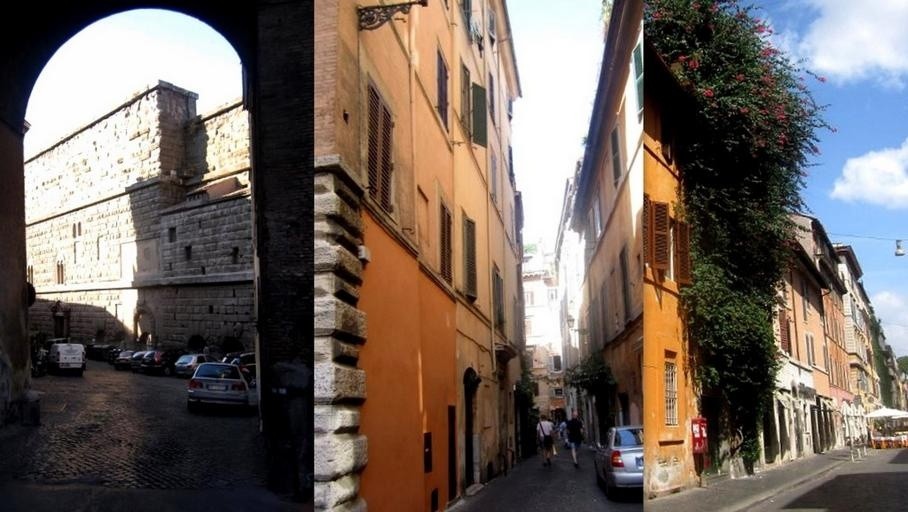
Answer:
[543, 434, 553, 448]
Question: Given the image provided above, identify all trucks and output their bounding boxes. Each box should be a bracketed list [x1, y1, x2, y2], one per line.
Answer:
[47, 343, 86, 376]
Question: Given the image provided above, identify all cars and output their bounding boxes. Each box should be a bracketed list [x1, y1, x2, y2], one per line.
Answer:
[186, 362, 250, 414]
[220, 351, 256, 379]
[87, 336, 151, 375]
[142, 346, 190, 376]
[173, 353, 223, 380]
[591, 425, 642, 500]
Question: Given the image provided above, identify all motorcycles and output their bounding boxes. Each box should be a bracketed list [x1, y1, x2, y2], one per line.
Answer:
[226, 359, 255, 391]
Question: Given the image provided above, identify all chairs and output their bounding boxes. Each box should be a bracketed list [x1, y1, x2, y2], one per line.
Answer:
[872, 436, 908, 449]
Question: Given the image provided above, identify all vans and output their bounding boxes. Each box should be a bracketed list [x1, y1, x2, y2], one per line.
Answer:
[44, 337, 69, 350]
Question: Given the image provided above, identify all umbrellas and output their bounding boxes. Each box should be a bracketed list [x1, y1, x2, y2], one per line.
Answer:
[863, 406, 908, 438]
[841, 400, 860, 462]
[888, 409, 908, 431]
[849, 400, 865, 460]
[857, 403, 868, 457]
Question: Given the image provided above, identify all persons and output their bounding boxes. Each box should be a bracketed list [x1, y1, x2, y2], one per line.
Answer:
[536, 409, 586, 467]
